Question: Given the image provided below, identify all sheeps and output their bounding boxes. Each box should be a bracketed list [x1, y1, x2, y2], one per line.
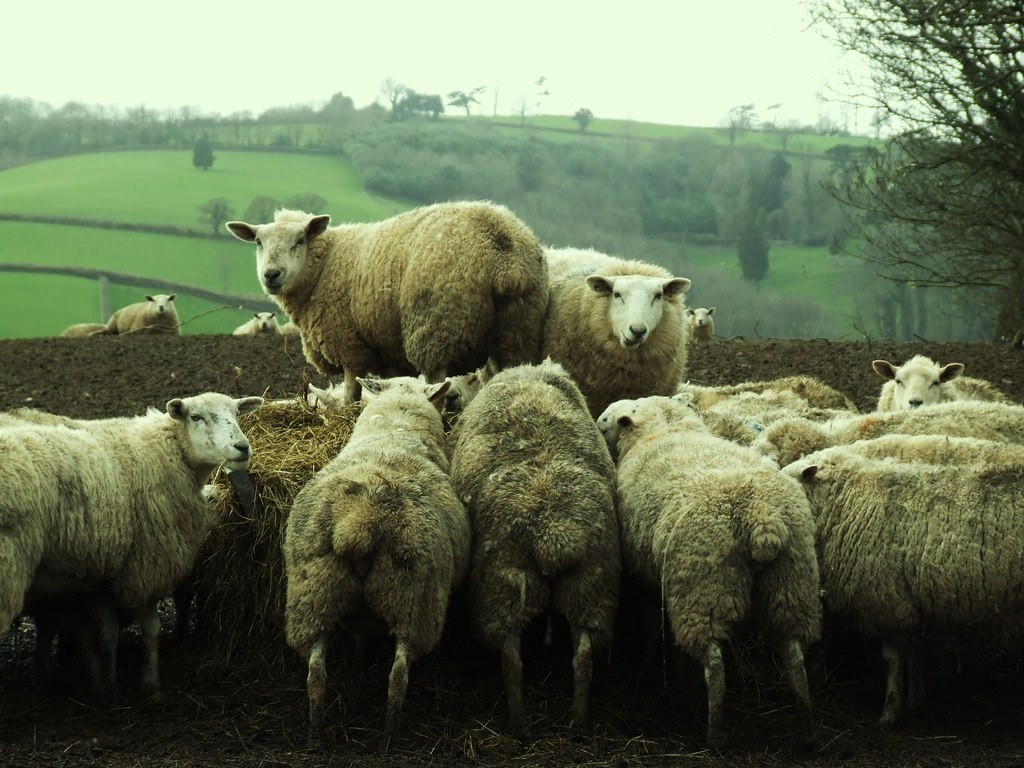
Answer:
[60, 293, 181, 335]
[686, 305, 716, 346]
[0, 392, 265, 710]
[284, 349, 1023, 768]
[223, 207, 692, 420]
